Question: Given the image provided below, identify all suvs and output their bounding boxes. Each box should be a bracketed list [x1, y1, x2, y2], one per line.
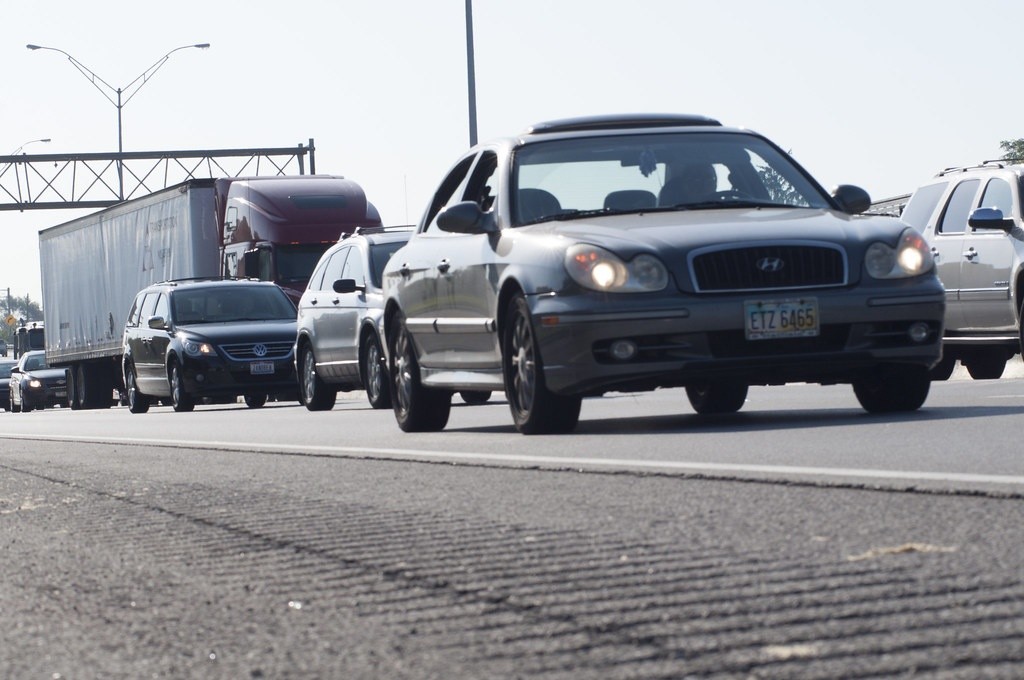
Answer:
[291, 215, 492, 412]
[899, 156, 1023, 382]
[120, 274, 300, 415]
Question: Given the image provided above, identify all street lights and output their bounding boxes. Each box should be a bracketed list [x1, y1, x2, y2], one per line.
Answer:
[25, 42, 212, 200]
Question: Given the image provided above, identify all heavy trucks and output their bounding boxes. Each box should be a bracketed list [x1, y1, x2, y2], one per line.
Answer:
[37, 175, 388, 410]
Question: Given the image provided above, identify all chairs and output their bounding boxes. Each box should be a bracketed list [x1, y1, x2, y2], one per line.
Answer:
[29, 359, 40, 369]
[515, 189, 562, 224]
[604, 191, 658, 211]
[659, 176, 688, 207]
[178, 299, 201, 322]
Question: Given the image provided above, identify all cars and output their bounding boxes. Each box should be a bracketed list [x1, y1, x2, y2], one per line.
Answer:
[0, 355, 19, 412]
[0, 339, 9, 358]
[382, 112, 945, 436]
[8, 349, 72, 414]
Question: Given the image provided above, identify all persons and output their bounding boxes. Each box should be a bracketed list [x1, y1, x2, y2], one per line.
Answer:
[682, 164, 717, 202]
